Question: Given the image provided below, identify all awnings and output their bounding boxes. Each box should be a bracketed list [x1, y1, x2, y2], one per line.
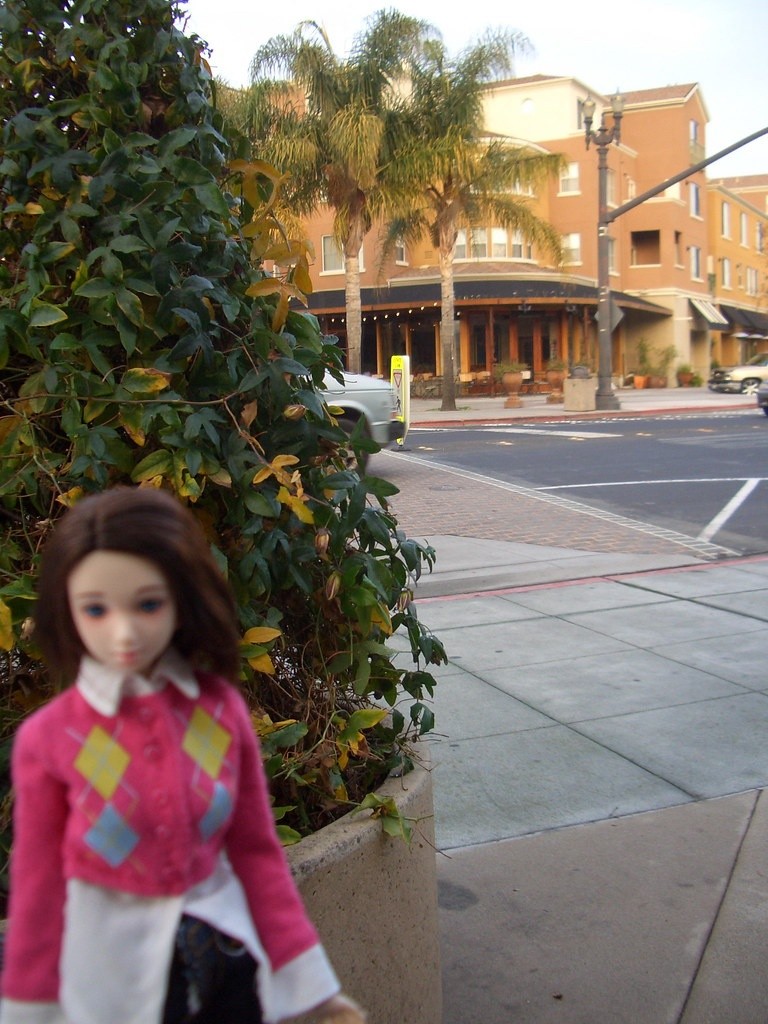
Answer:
[690, 298, 729, 330]
[720, 304, 768, 339]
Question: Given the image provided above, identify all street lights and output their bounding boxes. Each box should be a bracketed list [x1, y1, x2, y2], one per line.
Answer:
[581, 88, 623, 409]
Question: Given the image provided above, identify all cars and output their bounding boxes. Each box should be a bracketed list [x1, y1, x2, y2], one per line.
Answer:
[755, 377, 768, 416]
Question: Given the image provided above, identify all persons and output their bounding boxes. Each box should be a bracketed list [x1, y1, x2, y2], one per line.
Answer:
[0, 489, 365, 1024]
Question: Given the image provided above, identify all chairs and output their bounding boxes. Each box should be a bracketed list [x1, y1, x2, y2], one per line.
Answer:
[416, 371, 549, 398]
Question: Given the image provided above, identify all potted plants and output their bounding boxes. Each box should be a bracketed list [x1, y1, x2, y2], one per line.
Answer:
[0, 0, 444, 1024]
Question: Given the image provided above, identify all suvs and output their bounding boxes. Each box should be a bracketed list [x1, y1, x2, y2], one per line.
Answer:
[707, 352, 768, 395]
[308, 368, 404, 481]
